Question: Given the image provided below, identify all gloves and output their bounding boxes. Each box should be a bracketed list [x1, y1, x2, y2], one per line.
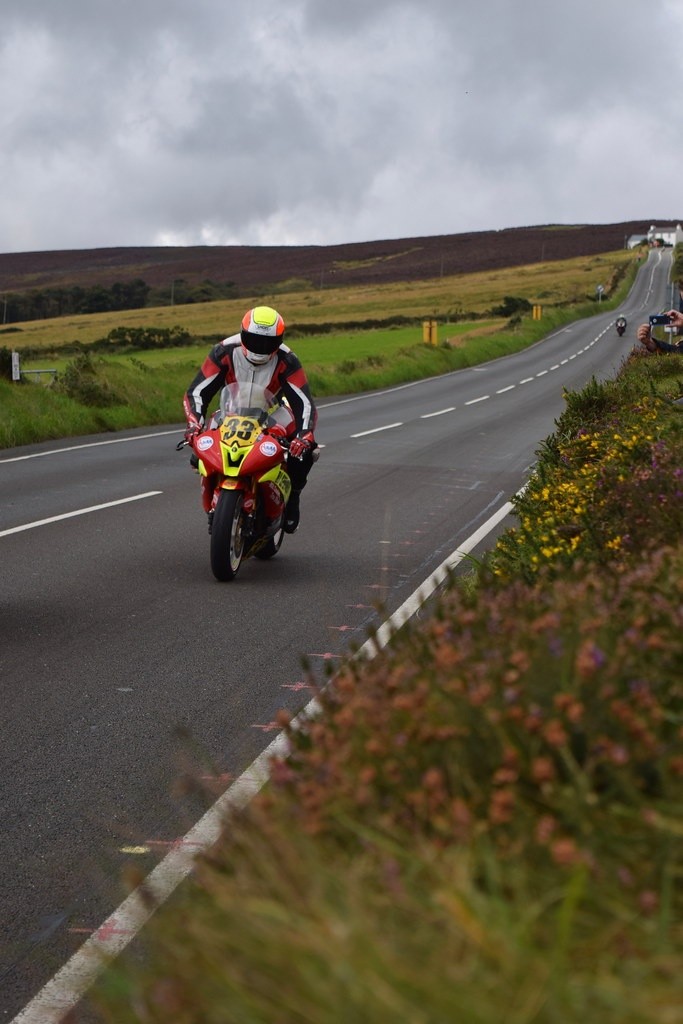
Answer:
[289, 438, 312, 458]
[185, 423, 201, 446]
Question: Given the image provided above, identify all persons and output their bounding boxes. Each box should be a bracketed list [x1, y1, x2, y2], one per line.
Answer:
[616, 311, 627, 335]
[181, 306, 320, 537]
[638, 309, 683, 353]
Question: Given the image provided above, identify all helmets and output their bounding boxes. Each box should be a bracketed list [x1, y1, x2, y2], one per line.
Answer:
[241, 306, 285, 364]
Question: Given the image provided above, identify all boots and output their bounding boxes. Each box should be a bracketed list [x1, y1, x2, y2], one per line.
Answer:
[190, 454, 199, 469]
[283, 479, 307, 533]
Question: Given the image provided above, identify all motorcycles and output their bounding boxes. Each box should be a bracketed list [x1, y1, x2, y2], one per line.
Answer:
[616, 318, 628, 337]
[188, 380, 321, 581]
